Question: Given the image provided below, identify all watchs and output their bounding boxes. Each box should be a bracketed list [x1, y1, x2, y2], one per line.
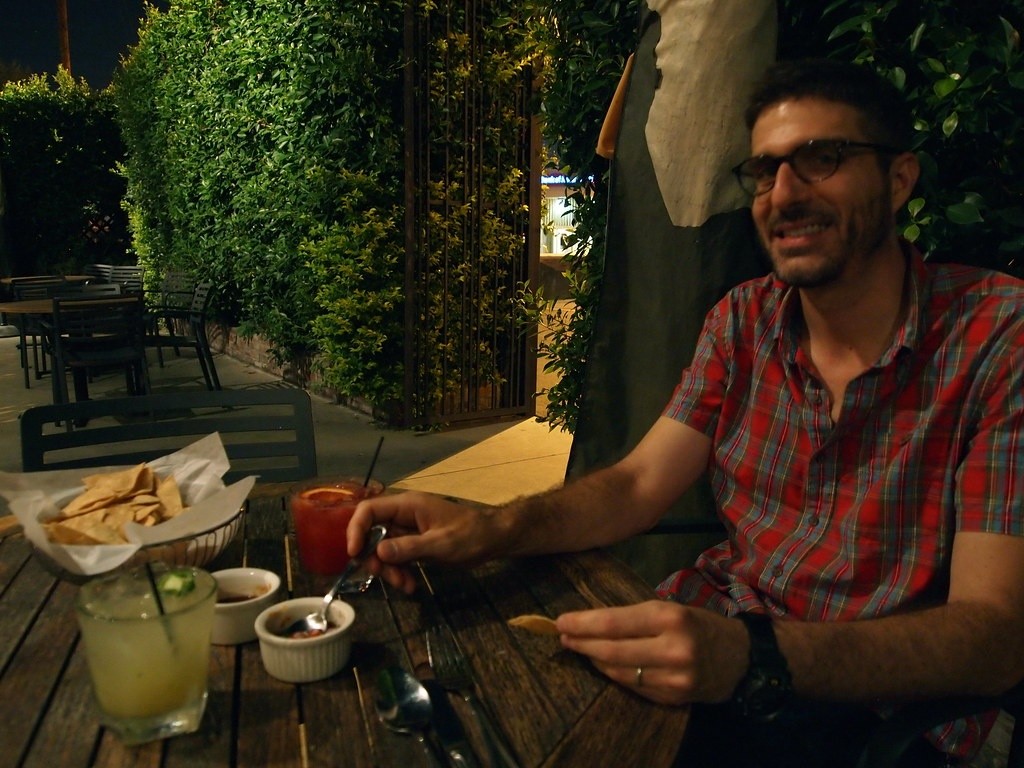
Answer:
[732, 609, 806, 718]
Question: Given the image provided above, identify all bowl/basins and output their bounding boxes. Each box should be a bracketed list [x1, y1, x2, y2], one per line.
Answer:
[254, 596, 356, 682]
[209, 567, 280, 644]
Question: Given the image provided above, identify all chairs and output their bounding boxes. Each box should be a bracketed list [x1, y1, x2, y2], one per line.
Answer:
[1, 262, 318, 489]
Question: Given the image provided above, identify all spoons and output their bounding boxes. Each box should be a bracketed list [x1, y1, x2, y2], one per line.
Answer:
[373, 668, 449, 768]
[285, 525, 387, 637]
[321, 573, 375, 592]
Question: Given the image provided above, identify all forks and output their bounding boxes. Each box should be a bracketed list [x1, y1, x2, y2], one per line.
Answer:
[424, 622, 523, 767]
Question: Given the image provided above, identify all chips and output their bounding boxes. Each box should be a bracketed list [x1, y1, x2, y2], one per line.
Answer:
[40, 462, 191, 547]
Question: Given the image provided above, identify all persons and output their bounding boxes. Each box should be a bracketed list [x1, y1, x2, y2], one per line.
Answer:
[349, 61, 1024, 768]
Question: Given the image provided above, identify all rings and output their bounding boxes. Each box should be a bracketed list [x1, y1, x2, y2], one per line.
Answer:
[634, 664, 646, 690]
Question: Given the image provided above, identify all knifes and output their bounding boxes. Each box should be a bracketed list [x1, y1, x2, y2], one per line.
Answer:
[414, 661, 481, 768]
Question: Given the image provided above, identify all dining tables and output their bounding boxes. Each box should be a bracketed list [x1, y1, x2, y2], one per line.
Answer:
[0, 483, 703, 768]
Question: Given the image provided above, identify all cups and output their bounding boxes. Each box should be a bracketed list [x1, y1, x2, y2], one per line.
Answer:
[289, 477, 385, 574]
[75, 562, 218, 747]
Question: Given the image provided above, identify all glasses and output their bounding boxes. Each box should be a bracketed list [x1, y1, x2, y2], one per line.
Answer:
[730, 136, 879, 197]
